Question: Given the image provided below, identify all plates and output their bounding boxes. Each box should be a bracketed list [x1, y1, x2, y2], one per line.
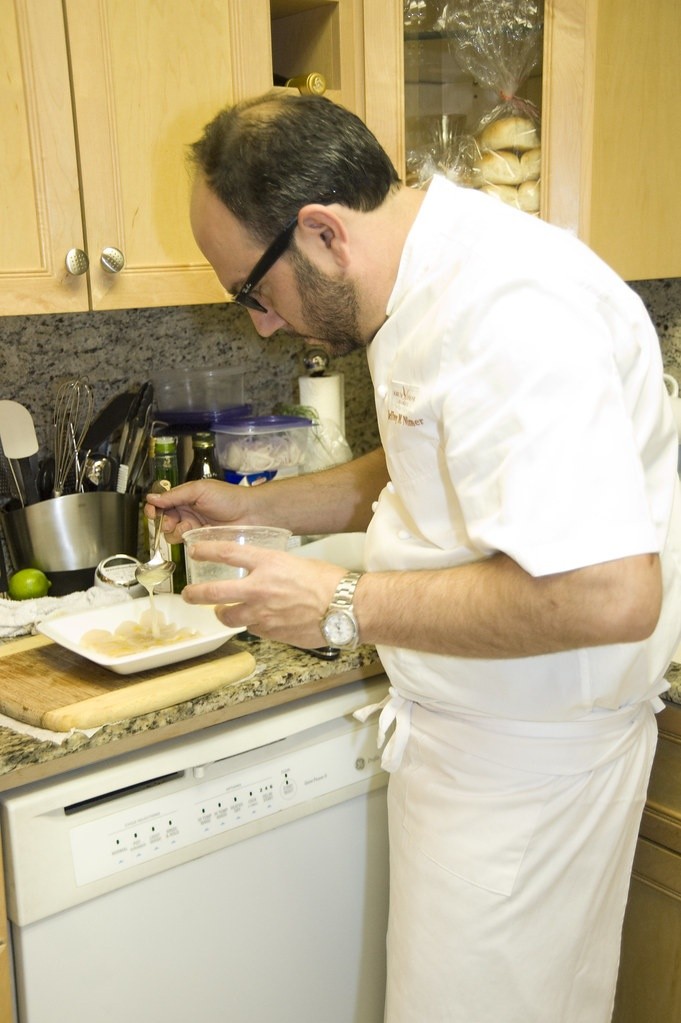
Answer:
[36, 592, 248, 674]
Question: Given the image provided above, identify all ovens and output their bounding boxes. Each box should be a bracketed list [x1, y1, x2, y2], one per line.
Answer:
[0, 673, 385, 1023]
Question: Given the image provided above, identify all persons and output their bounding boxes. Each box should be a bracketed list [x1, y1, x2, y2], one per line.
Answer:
[144, 87, 681, 1023]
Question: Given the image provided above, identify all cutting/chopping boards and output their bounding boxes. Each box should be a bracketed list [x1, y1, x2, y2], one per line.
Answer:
[0, 633, 256, 734]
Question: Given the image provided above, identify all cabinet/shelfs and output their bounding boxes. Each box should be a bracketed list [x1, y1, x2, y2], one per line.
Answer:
[609, 698, 681, 1023]
[589, 0, 681, 282]
[244, 0, 597, 251]
[0, 0, 244, 317]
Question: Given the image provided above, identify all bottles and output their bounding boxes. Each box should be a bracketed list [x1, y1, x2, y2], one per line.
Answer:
[138, 431, 227, 594]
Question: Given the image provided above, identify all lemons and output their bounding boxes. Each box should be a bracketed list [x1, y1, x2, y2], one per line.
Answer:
[8, 568, 52, 601]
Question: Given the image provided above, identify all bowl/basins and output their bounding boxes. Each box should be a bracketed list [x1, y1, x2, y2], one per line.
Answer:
[180, 525, 293, 607]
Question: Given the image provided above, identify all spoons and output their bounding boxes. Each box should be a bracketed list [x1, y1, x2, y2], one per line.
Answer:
[134, 480, 176, 588]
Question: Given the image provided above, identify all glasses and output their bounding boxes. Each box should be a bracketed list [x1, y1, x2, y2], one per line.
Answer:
[232, 188, 336, 313]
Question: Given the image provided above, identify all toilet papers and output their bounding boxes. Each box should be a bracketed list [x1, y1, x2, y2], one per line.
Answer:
[299, 370, 345, 439]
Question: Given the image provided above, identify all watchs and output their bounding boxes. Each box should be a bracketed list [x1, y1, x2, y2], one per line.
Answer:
[320, 571, 366, 652]
